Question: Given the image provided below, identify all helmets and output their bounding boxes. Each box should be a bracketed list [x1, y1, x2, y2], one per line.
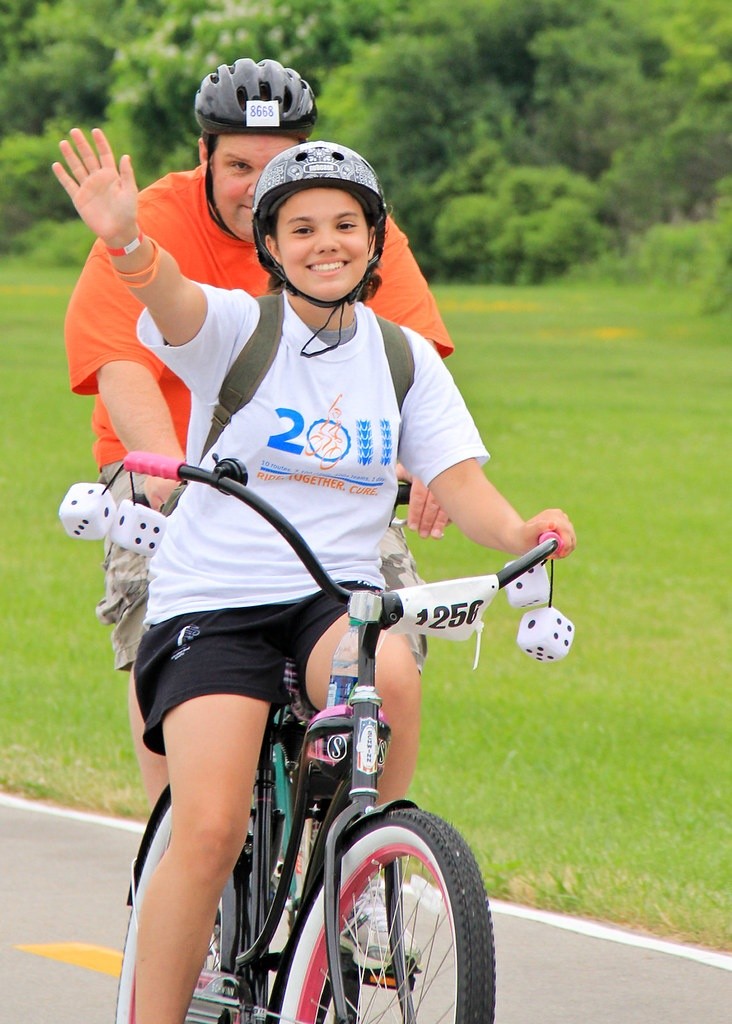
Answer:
[251, 140, 389, 226]
[192, 55, 320, 139]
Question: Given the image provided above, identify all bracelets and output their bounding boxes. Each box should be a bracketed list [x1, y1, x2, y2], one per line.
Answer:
[107, 237, 161, 277]
[111, 260, 158, 289]
[101, 228, 144, 257]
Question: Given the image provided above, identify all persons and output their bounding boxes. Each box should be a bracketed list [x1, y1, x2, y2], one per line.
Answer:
[45, 128, 577, 1023]
[65, 58, 455, 805]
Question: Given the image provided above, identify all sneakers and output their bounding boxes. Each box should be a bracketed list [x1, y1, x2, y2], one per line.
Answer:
[339, 875, 425, 977]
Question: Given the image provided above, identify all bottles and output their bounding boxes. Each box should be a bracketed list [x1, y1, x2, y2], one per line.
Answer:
[325, 619, 377, 707]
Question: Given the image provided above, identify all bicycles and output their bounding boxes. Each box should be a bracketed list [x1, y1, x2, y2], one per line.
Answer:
[115, 442, 571, 1024]
